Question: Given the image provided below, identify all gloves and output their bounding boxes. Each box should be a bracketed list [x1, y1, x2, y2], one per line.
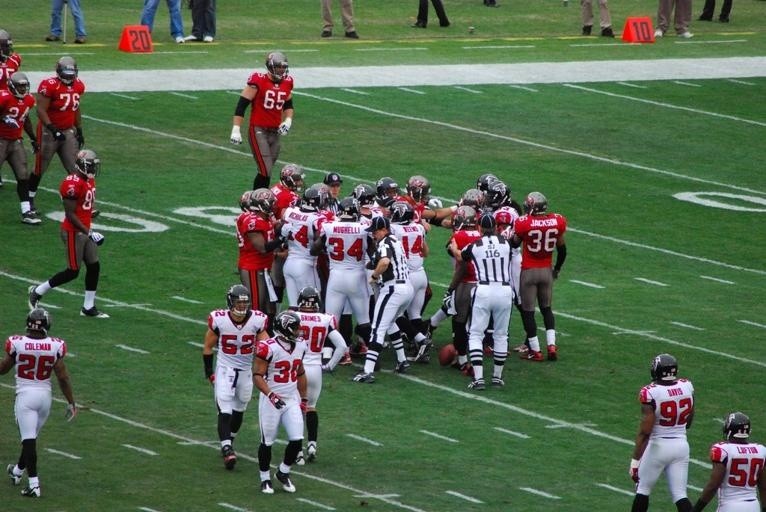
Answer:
[65, 404, 76, 422]
[445, 288, 456, 310]
[427, 197, 444, 207]
[231, 132, 242, 145]
[277, 122, 290, 136]
[88, 229, 104, 246]
[30, 127, 88, 153]
[629, 468, 640, 483]
[209, 373, 309, 416]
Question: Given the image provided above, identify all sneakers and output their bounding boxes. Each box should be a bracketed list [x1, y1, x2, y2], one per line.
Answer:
[7, 464, 41, 498]
[28, 285, 42, 310]
[185, 35, 197, 40]
[80, 305, 110, 319]
[222, 437, 317, 494]
[204, 36, 213, 43]
[21, 208, 42, 224]
[176, 36, 185, 43]
[322, 320, 562, 391]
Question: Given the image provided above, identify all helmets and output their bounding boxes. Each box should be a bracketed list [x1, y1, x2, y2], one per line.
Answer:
[265, 52, 289, 80]
[227, 284, 322, 342]
[722, 412, 752, 442]
[451, 173, 547, 229]
[651, 354, 679, 382]
[25, 307, 51, 335]
[240, 164, 430, 232]
[0, 30, 80, 100]
[73, 149, 101, 179]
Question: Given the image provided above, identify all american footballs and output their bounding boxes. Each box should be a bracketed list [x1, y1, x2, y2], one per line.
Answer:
[439, 344, 455, 366]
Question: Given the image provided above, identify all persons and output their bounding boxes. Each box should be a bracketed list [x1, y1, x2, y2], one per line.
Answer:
[696, 0, 733, 23]
[654, 0, 694, 39]
[141, 0, 185, 43]
[410, 0, 451, 28]
[0, 308, 76, 497]
[0, 0, 100, 225]
[27, 149, 108, 318]
[230, 52, 294, 191]
[689, 411, 766, 512]
[235, 166, 568, 390]
[629, 353, 694, 512]
[203, 286, 347, 492]
[184, 0, 216, 43]
[320, 0, 359, 39]
[580, 0, 615, 38]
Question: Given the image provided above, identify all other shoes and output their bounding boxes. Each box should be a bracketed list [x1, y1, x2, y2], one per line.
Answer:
[719, 17, 727, 22]
[46, 35, 60, 41]
[603, 29, 614, 37]
[321, 31, 331, 37]
[654, 29, 662, 37]
[411, 22, 426, 28]
[345, 31, 358, 39]
[677, 31, 693, 39]
[582, 26, 591, 35]
[75, 36, 85, 43]
[697, 17, 712, 22]
